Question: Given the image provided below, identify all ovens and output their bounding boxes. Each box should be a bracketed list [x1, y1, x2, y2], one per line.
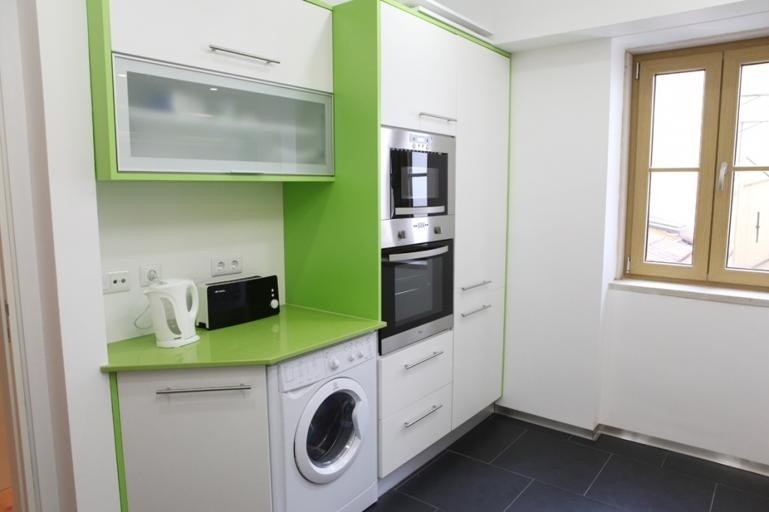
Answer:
[380, 124, 456, 249]
[378, 236, 454, 357]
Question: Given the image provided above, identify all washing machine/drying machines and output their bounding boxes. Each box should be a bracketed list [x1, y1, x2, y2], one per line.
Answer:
[266, 333, 378, 512]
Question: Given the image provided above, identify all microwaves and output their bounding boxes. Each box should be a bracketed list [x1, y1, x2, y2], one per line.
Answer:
[196, 270, 280, 331]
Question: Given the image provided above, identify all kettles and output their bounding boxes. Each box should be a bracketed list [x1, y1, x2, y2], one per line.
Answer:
[131, 274, 200, 348]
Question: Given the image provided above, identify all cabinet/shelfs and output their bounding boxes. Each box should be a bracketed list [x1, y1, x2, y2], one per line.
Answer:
[453, 29, 513, 448]
[110, 365, 275, 512]
[332, 0, 458, 136]
[377, 328, 453, 499]
[107, 0, 336, 183]
